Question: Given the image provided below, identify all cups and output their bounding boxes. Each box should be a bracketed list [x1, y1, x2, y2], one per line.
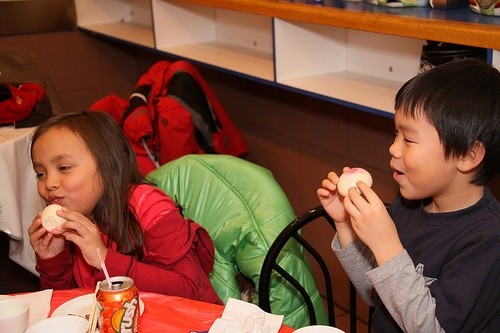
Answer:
[291, 324, 345, 333]
[26, 315, 91, 333]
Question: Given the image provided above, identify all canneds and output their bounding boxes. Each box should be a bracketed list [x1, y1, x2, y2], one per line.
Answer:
[95, 276, 142, 333]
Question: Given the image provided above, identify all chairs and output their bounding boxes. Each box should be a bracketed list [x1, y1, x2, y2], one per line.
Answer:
[143, 152, 330, 330]
[258, 202, 392, 333]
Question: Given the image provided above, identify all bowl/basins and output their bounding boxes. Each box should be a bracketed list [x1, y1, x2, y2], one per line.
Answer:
[0, 299, 30, 333]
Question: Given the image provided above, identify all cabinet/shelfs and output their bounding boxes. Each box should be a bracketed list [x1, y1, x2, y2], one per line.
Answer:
[75, 0, 500, 120]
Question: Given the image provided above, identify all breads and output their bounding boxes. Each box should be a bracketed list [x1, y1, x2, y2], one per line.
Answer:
[41, 203, 70, 234]
[337, 167, 372, 197]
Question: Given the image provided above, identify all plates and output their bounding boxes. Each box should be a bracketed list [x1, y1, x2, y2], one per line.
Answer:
[51, 292, 146, 323]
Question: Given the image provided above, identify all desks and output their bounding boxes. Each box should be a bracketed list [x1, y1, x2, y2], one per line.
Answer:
[0, 126, 46, 277]
[0, 289, 294, 333]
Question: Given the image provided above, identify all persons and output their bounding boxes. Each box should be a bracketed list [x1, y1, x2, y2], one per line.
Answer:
[316, 59, 500, 333]
[28, 111, 223, 305]
